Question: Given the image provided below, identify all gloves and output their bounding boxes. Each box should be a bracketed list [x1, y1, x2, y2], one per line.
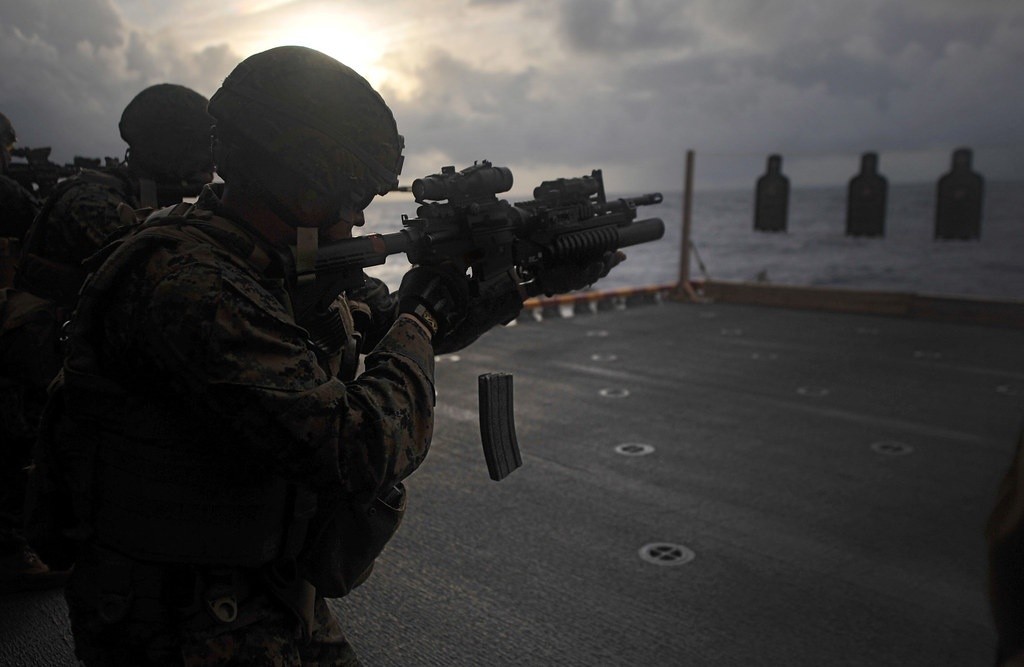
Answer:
[532, 243, 626, 298]
[396, 265, 470, 336]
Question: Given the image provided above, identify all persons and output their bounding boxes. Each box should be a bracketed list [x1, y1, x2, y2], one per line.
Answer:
[0, 45, 625, 667]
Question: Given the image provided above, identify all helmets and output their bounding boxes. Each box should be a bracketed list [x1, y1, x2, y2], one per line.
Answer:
[207, 49, 406, 212]
[121, 84, 219, 166]
[0, 113, 16, 149]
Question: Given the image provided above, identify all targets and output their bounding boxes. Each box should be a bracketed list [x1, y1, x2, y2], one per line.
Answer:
[846, 152, 888, 239]
[935, 146, 985, 241]
[753, 153, 791, 232]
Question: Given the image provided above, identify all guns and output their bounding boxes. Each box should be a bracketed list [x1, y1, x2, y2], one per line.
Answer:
[263, 160, 666, 304]
[10, 145, 123, 189]
